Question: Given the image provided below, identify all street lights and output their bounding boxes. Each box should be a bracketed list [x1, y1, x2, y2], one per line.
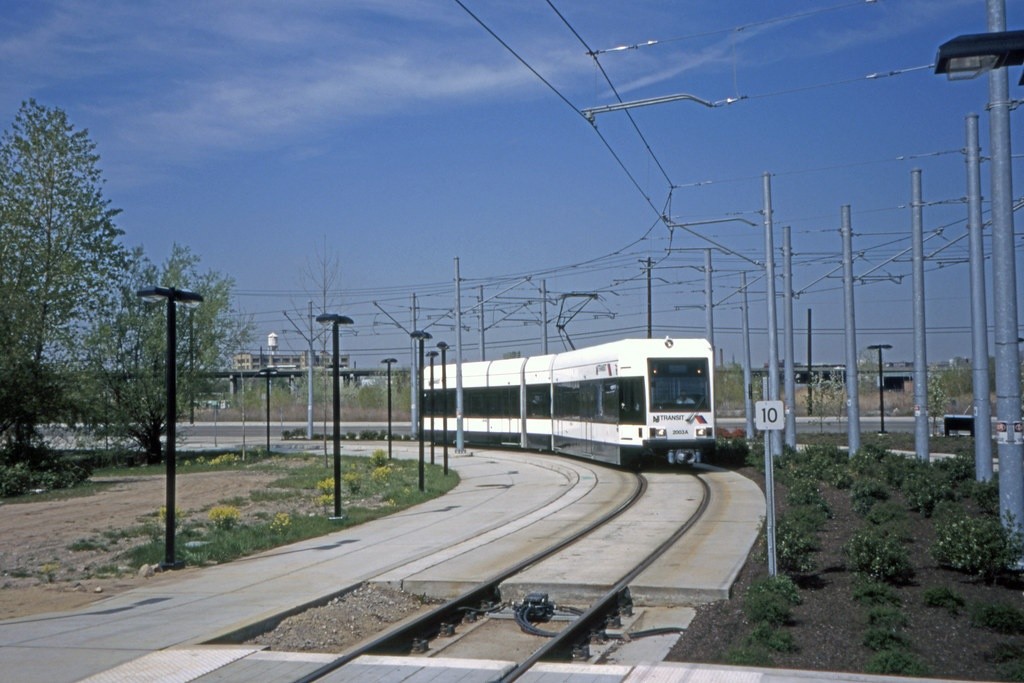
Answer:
[867, 344, 892, 434]
[410, 330, 433, 491]
[381, 358, 397, 460]
[437, 342, 450, 475]
[135, 286, 205, 570]
[260, 368, 278, 452]
[425, 351, 439, 464]
[316, 312, 354, 518]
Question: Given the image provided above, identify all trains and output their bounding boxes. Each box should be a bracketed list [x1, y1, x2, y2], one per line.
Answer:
[423, 337, 715, 465]
